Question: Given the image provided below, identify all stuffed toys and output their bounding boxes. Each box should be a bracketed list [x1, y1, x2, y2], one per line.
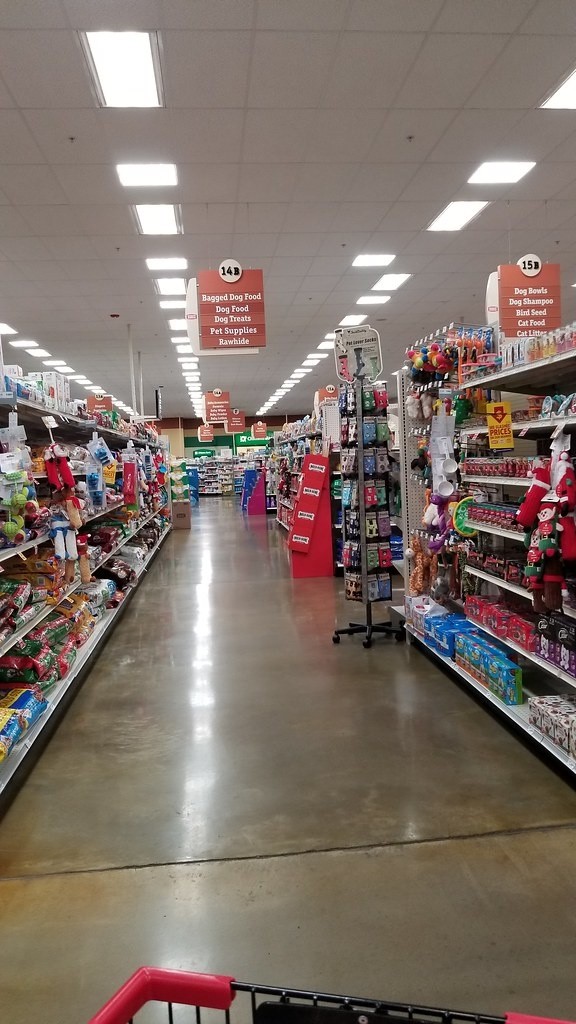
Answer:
[406, 538, 461, 605]
[43, 442, 91, 583]
[514, 452, 576, 613]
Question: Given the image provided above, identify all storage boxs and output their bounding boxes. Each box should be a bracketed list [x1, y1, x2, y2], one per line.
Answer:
[0, 365, 71, 414]
[456, 595, 576, 760]
[172, 497, 192, 530]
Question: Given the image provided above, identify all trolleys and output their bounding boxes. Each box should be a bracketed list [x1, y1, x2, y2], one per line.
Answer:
[88, 967, 576, 1024]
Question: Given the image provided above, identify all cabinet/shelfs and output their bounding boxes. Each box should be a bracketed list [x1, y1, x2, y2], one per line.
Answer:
[240, 381, 398, 575]
[0, 392, 172, 821]
[180, 459, 236, 495]
[390, 336, 576, 775]
